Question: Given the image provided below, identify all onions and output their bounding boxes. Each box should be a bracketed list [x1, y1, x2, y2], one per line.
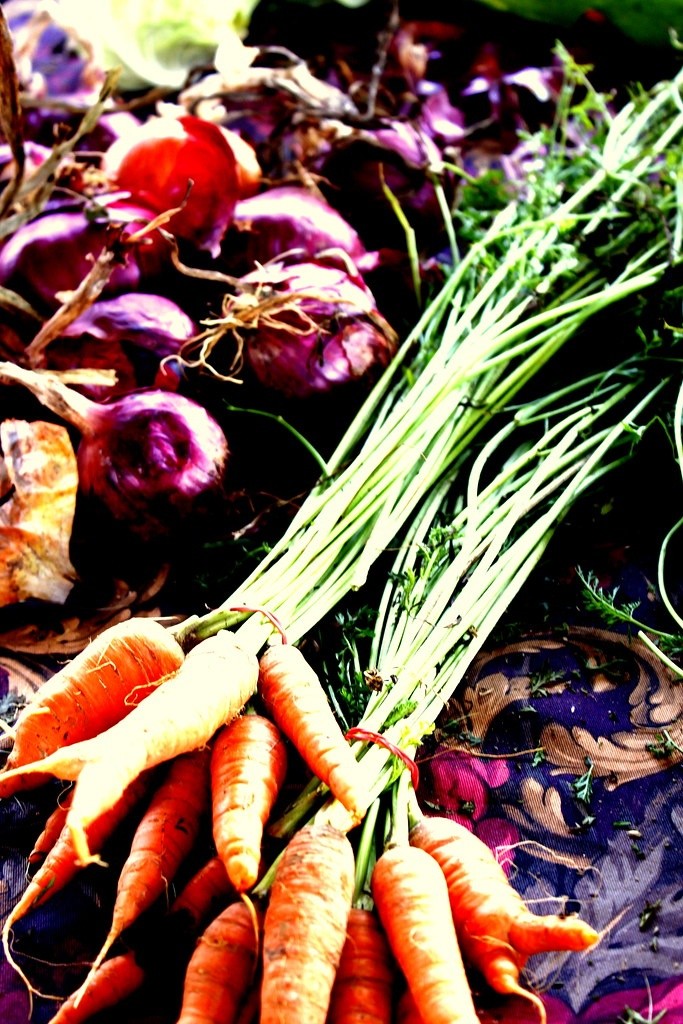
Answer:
[0, 53, 454, 527]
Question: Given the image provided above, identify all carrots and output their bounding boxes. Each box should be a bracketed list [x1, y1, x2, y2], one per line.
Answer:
[0, 616, 600, 1024]
